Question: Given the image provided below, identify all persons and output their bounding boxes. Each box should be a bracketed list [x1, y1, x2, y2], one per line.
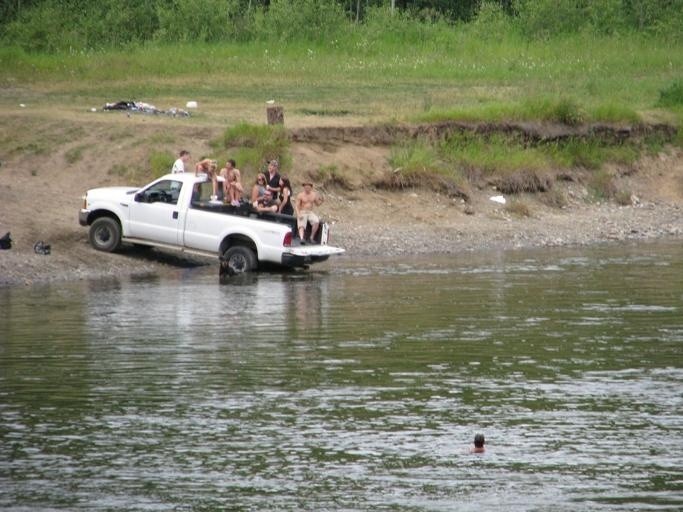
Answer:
[168, 151, 325, 246]
[471, 432, 487, 453]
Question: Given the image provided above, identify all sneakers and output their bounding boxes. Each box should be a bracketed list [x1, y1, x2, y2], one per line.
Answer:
[300, 239, 316, 245]
[231, 200, 240, 207]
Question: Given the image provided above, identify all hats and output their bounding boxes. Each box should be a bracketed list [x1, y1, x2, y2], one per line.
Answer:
[302, 180, 313, 187]
[266, 160, 278, 167]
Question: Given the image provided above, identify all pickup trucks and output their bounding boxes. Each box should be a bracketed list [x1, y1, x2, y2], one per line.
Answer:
[79, 172, 345, 275]
